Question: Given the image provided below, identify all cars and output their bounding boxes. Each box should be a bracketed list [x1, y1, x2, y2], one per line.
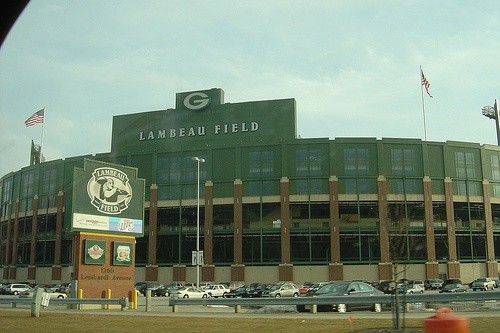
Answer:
[469, 277, 500, 291]
[135, 280, 300, 298]
[0, 283, 71, 300]
[296, 282, 385, 313]
[297, 278, 467, 295]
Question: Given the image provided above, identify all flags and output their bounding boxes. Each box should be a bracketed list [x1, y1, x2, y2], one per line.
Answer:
[421, 69, 433, 98]
[25, 108, 44, 127]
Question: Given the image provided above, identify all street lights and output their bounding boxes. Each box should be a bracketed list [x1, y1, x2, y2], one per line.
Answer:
[192, 157, 206, 288]
